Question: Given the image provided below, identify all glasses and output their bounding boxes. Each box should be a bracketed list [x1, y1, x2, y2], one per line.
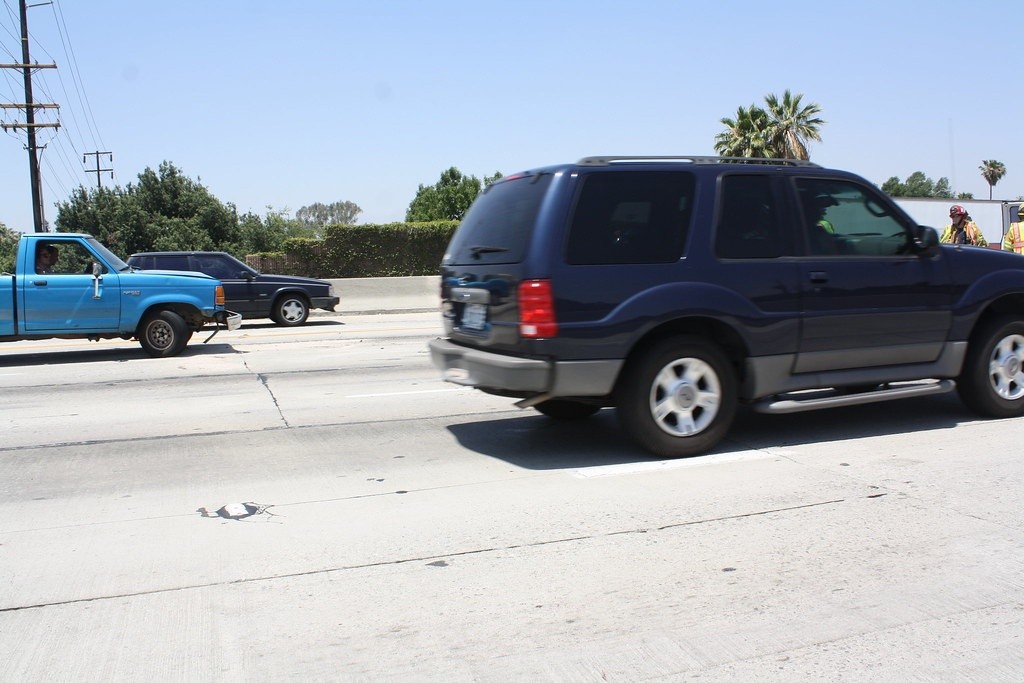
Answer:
[949, 214, 959, 217]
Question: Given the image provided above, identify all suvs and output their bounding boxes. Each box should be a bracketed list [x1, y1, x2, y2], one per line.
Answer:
[124, 250, 341, 327]
[425, 155, 1023, 458]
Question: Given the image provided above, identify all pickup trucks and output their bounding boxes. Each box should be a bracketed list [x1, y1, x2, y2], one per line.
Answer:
[0, 232, 244, 359]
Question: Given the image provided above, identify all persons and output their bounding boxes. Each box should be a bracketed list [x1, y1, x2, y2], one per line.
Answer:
[36, 246, 59, 273]
[1004, 204, 1024, 255]
[817, 208, 834, 235]
[939, 205, 987, 247]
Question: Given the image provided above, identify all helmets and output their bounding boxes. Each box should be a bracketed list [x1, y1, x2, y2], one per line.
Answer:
[1017, 203, 1024, 215]
[950, 205, 965, 214]
